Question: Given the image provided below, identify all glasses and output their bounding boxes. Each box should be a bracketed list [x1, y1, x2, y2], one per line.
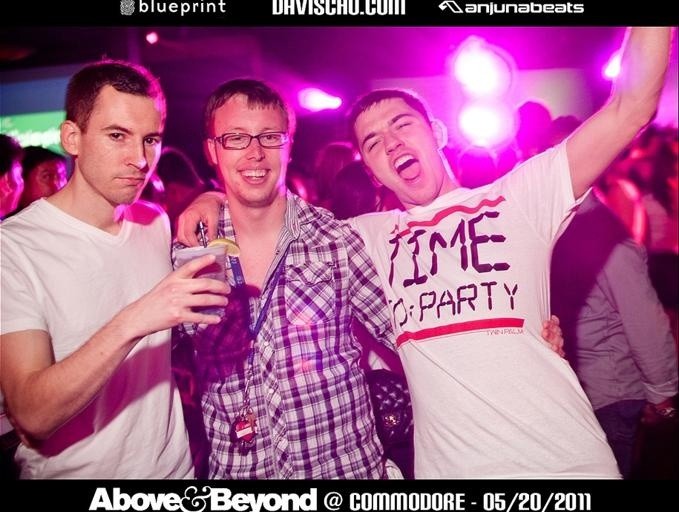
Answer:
[213, 131, 289, 150]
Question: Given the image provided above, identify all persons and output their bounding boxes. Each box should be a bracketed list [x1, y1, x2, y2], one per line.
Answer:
[1, 27, 677, 479]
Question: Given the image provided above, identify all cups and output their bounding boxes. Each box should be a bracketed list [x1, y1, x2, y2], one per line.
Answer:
[174, 244, 229, 319]
